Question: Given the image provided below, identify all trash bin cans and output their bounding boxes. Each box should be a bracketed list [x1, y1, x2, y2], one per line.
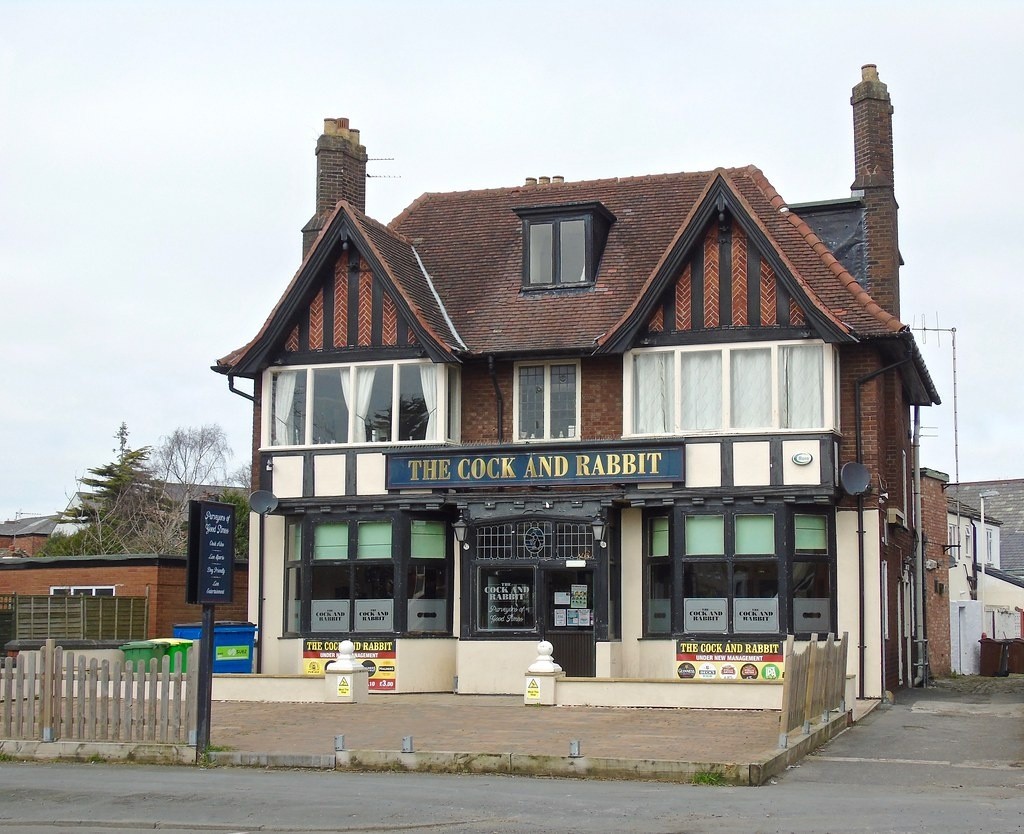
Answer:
[119, 639, 170, 673]
[149, 637, 194, 674]
[169, 621, 259, 673]
[998, 638, 1013, 677]
[978, 637, 1004, 677]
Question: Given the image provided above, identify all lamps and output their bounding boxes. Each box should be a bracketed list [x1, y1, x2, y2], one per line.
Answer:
[590, 510, 617, 541]
[450, 508, 471, 541]
